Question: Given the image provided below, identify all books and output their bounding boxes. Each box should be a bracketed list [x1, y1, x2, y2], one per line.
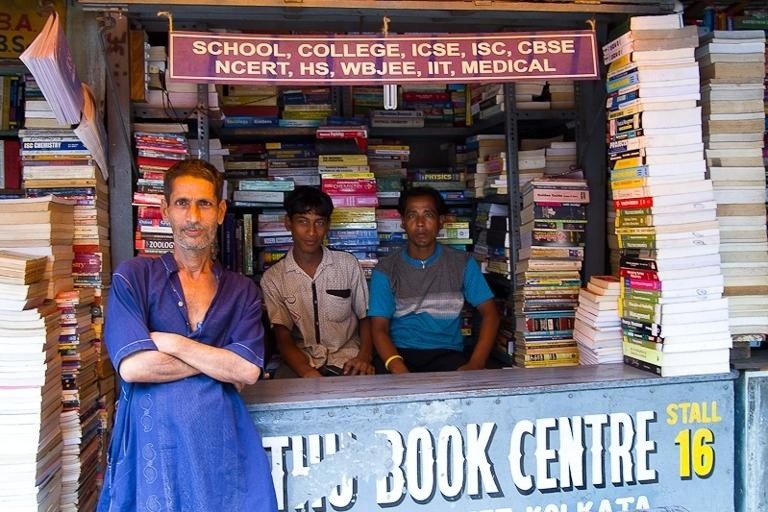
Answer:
[513, 136, 590, 367]
[602, 5, 768, 379]
[266, 129, 379, 195]
[136, 122, 229, 259]
[148, 30, 577, 128]
[572, 275, 623, 366]
[466, 132, 512, 280]
[368, 131, 411, 256]
[0, 8, 113, 512]
[322, 196, 379, 280]
[219, 130, 296, 275]
[412, 133, 474, 254]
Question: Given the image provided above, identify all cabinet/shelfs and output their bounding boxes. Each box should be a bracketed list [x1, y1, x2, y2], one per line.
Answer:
[0, 0, 79, 199]
[730, 364, 767, 511]
[474, 27, 606, 364]
[106, 10, 225, 271]
[227, 31, 471, 308]
[242, 370, 740, 511]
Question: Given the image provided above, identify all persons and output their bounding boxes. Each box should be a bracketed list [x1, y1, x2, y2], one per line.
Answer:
[366, 187, 502, 374]
[260, 186, 374, 379]
[95, 158, 279, 511]
